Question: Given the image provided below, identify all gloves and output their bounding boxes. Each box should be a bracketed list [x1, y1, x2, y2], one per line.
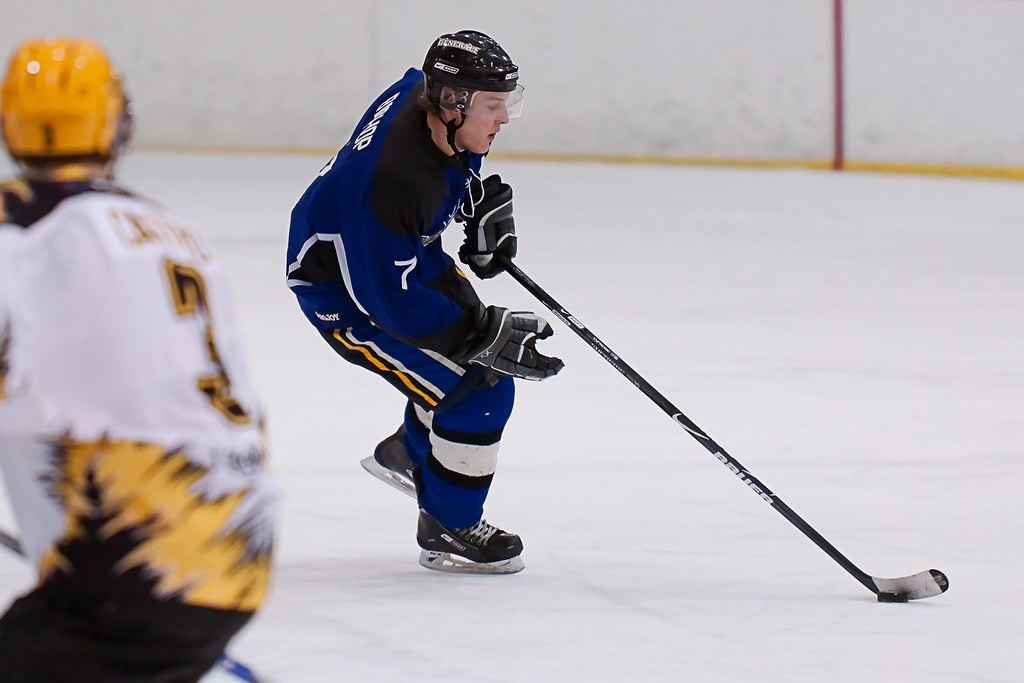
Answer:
[461, 305, 565, 382]
[453, 174, 518, 280]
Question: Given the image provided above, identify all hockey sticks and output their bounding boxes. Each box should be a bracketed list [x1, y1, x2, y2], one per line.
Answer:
[504, 260, 952, 604]
[0, 529, 269, 683]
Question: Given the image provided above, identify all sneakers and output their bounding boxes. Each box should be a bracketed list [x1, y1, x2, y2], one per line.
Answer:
[412, 463, 526, 574]
[360, 422, 418, 501]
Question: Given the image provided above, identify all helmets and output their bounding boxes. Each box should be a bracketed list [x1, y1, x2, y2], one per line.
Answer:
[421, 29, 519, 92]
[0, 37, 132, 157]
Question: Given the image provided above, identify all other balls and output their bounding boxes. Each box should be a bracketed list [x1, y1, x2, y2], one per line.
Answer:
[877, 591, 908, 603]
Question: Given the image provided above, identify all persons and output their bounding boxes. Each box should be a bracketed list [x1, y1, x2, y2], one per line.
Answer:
[287, 27, 567, 576]
[0, 34, 278, 683]
